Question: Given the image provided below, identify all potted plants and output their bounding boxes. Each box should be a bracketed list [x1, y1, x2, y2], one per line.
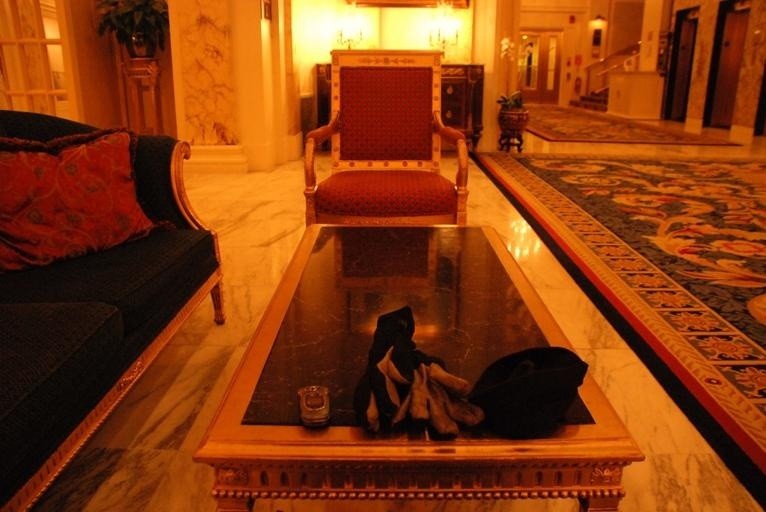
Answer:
[95, 0, 169, 58]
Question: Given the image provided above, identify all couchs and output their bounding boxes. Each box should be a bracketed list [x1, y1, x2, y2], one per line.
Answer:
[0, 110, 227, 511]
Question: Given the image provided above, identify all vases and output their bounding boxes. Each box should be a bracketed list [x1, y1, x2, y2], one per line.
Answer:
[495, 107, 530, 138]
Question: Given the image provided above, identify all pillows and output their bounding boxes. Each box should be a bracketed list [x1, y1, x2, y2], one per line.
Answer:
[0, 132, 156, 271]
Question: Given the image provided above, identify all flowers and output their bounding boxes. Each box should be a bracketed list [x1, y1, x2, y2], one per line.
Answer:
[496, 36, 534, 107]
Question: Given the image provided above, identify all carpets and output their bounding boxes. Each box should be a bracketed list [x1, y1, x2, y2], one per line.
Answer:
[468, 152, 766, 511]
[524, 103, 744, 146]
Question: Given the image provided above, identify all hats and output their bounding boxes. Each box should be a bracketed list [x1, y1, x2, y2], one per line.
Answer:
[470, 346, 588, 438]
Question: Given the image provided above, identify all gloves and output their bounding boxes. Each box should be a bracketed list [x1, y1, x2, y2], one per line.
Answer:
[386, 349, 485, 439]
[351, 305, 417, 438]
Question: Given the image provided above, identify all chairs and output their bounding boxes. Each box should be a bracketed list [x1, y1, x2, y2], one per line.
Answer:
[303, 49, 470, 225]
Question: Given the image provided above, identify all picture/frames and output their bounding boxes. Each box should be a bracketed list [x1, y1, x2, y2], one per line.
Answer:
[346, 250, 461, 341]
[264, 0, 272, 19]
[346, 0, 470, 9]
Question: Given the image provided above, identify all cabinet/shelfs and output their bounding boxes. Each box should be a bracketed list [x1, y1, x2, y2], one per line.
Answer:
[315, 63, 484, 152]
[606, 71, 661, 120]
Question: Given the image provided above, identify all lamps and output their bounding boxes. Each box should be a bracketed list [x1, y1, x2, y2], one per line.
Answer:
[336, 29, 362, 51]
[429, 27, 458, 64]
